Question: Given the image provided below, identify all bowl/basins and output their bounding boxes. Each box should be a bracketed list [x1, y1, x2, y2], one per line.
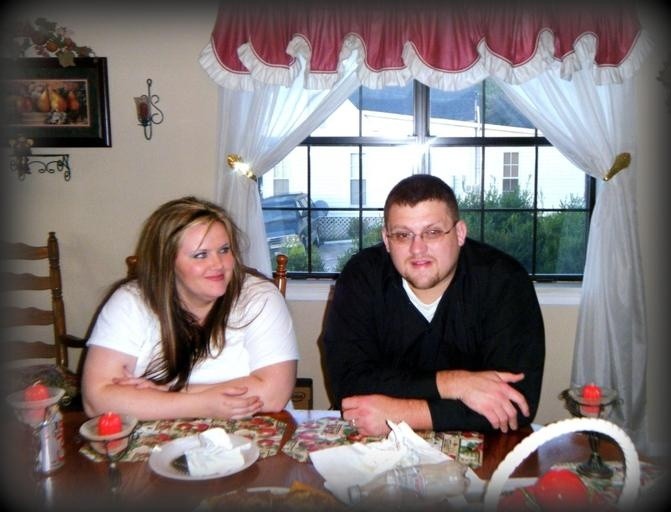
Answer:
[566, 386, 616, 418]
[80, 413, 137, 454]
[7, 384, 65, 425]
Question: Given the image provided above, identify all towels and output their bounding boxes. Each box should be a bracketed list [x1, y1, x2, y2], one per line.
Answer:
[182, 427, 246, 475]
[307, 418, 485, 508]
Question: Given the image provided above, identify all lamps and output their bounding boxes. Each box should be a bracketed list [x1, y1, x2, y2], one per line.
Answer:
[134, 79, 164, 140]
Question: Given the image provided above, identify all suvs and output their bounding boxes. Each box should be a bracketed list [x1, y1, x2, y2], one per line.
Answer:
[262, 193, 322, 259]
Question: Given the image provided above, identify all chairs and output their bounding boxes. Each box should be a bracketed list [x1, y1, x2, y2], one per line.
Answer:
[125, 254, 289, 297]
[1, 232, 85, 377]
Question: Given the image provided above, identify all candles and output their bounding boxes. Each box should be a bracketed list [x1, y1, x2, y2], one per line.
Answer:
[97, 410, 124, 450]
[583, 383, 601, 413]
[23, 380, 50, 422]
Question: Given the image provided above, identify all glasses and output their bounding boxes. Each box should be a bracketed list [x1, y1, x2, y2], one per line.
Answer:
[386, 220, 458, 242]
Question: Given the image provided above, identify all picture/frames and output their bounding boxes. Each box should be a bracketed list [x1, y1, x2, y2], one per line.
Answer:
[0, 57, 112, 148]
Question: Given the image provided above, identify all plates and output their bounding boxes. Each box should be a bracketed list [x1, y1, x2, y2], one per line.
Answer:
[147, 433, 261, 481]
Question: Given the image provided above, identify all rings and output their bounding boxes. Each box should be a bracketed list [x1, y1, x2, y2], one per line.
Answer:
[352, 418, 357, 430]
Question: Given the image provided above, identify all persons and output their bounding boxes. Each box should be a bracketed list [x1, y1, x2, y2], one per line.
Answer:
[81, 196, 301, 419]
[317, 173, 545, 436]
